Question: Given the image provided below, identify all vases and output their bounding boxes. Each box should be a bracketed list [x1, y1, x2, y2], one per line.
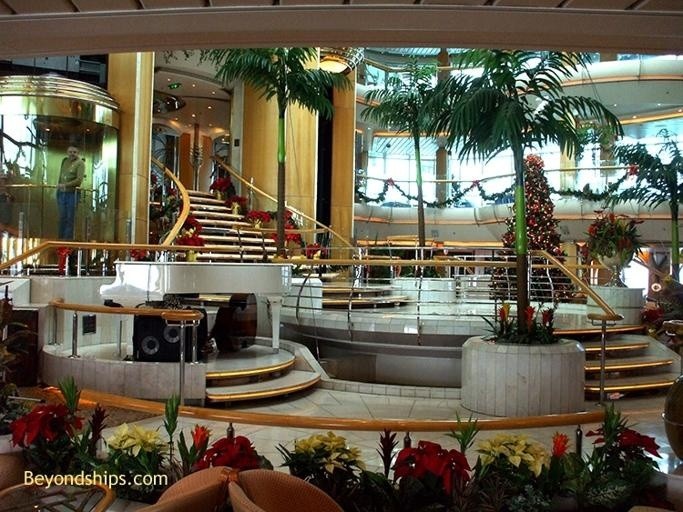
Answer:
[216, 191, 225, 200]
[597, 250, 631, 288]
[256, 220, 263, 228]
[185, 250, 195, 262]
[233, 206, 240, 215]
[169, 196, 176, 202]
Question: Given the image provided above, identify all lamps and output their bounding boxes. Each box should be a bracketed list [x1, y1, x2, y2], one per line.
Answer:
[321, 48, 365, 74]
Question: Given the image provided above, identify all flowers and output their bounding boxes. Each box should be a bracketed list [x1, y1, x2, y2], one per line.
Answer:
[225, 196, 248, 207]
[167, 189, 177, 195]
[245, 210, 271, 221]
[173, 236, 205, 249]
[586, 209, 651, 258]
[209, 178, 232, 190]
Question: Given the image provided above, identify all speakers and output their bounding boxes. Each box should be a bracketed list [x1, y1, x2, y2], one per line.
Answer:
[133, 309, 208, 363]
[4, 308, 39, 387]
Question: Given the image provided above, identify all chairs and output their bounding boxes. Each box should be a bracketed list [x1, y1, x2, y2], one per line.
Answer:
[231, 468, 343, 512]
[133, 465, 230, 512]
[3, 476, 115, 512]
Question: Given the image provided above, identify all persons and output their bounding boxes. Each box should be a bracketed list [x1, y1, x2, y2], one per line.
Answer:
[56, 145, 85, 240]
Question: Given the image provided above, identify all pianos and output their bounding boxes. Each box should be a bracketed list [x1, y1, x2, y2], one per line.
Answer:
[99, 260, 294, 358]
